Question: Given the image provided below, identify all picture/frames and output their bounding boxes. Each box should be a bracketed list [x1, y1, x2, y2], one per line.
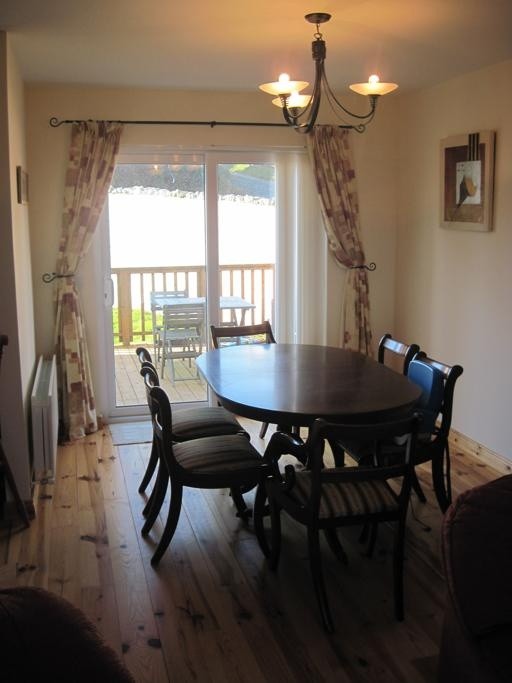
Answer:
[17, 163, 33, 206]
[436, 130, 496, 232]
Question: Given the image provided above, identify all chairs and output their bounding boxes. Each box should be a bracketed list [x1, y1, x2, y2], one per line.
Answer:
[149, 288, 194, 366]
[207, 320, 287, 438]
[0, 586, 138, 682]
[157, 304, 209, 385]
[139, 365, 275, 567]
[307, 333, 420, 467]
[334, 350, 464, 559]
[441, 475, 512, 683]
[267, 407, 424, 633]
[135, 346, 246, 515]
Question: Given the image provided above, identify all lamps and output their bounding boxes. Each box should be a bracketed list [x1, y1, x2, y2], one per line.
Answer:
[259, 11, 399, 134]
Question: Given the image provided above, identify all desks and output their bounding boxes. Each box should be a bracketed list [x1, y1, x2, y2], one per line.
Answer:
[154, 295, 256, 370]
[195, 343, 424, 557]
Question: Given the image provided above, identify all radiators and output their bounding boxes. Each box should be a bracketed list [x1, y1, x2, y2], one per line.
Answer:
[30, 353, 61, 481]
